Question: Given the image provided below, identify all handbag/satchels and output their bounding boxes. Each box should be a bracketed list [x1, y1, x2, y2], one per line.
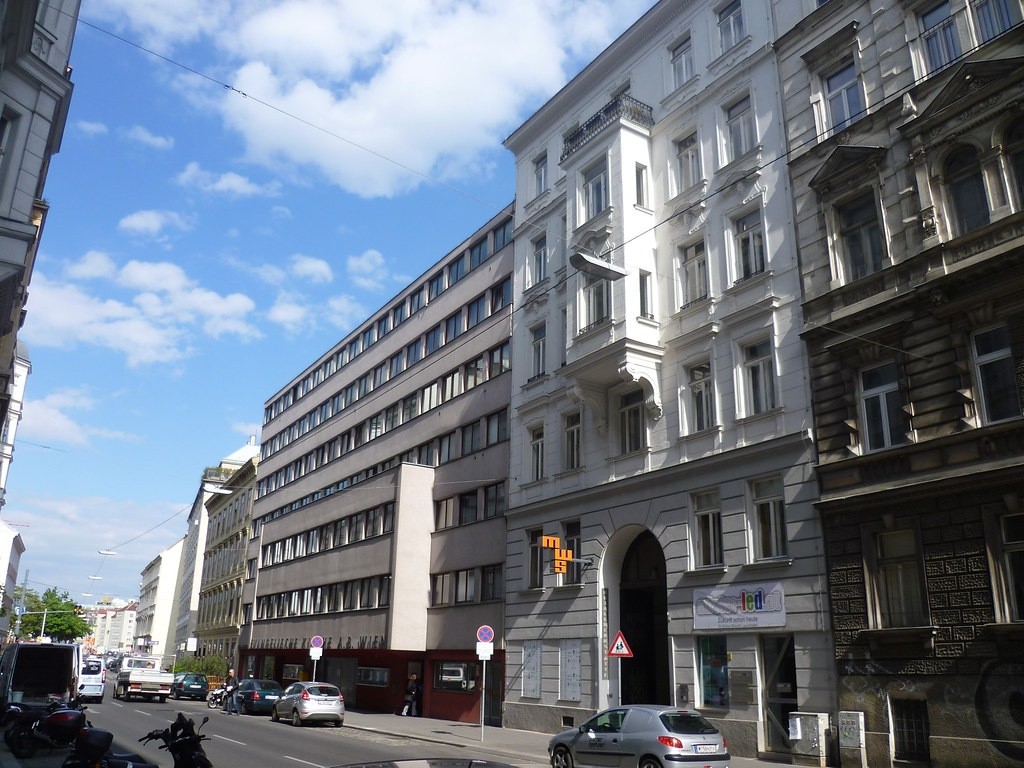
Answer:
[404, 692, 414, 702]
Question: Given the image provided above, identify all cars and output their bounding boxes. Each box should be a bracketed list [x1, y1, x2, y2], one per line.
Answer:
[547, 704, 731, 768]
[222, 679, 286, 714]
[271, 681, 345, 728]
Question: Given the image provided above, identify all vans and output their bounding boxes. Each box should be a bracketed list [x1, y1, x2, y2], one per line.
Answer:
[72, 656, 107, 703]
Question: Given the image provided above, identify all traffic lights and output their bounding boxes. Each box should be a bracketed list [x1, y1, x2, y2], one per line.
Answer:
[74, 605, 78, 614]
[78, 605, 82, 615]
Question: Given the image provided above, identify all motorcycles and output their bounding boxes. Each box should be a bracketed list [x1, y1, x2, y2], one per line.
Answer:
[207, 685, 228, 709]
[1, 682, 216, 768]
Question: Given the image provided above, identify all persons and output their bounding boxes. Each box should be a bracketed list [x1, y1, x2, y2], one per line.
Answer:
[401, 674, 420, 717]
[225, 669, 240, 716]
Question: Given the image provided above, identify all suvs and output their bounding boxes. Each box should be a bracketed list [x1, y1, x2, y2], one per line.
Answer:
[168, 673, 209, 701]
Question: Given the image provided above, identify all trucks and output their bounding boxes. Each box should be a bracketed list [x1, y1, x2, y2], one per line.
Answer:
[1, 640, 81, 709]
[113, 656, 174, 701]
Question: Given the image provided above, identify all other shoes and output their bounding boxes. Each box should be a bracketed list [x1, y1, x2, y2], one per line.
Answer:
[227, 712, 233, 715]
[236, 712, 241, 716]
